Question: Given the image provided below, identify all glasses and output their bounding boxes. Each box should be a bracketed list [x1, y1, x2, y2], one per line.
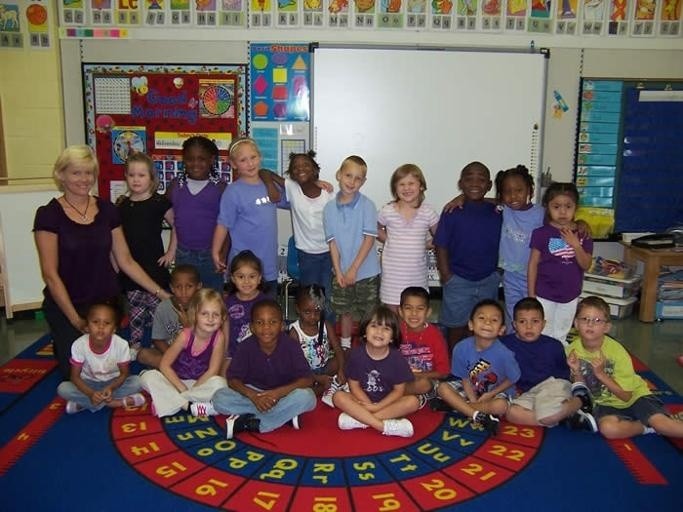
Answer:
[580, 316, 601, 325]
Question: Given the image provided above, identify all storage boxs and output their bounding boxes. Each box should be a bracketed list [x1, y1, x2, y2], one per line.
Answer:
[583, 271, 643, 300]
[579, 293, 638, 320]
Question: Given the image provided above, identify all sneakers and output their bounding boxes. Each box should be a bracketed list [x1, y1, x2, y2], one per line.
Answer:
[191, 402, 219, 416]
[430, 398, 451, 411]
[322, 383, 342, 408]
[66, 401, 84, 414]
[123, 394, 145, 408]
[382, 419, 414, 437]
[571, 382, 598, 432]
[227, 414, 248, 439]
[285, 416, 300, 430]
[338, 412, 369, 430]
[484, 413, 499, 435]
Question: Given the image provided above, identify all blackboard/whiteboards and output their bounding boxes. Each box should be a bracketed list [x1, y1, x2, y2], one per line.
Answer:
[308, 42, 550, 232]
[0, 183, 64, 311]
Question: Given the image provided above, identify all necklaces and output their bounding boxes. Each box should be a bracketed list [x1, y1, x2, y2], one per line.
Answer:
[63, 195, 90, 222]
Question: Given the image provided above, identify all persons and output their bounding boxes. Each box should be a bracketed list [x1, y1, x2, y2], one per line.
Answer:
[57, 304, 145, 413]
[33, 144, 174, 375]
[566, 297, 683, 439]
[110, 152, 177, 361]
[376, 164, 440, 313]
[142, 249, 347, 440]
[434, 161, 593, 358]
[323, 156, 379, 351]
[333, 286, 450, 437]
[439, 297, 598, 433]
[165, 135, 335, 315]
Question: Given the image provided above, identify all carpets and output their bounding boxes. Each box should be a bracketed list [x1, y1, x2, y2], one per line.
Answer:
[0, 325, 682, 511]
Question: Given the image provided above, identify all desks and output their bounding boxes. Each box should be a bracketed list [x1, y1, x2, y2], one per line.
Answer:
[619, 240, 683, 323]
